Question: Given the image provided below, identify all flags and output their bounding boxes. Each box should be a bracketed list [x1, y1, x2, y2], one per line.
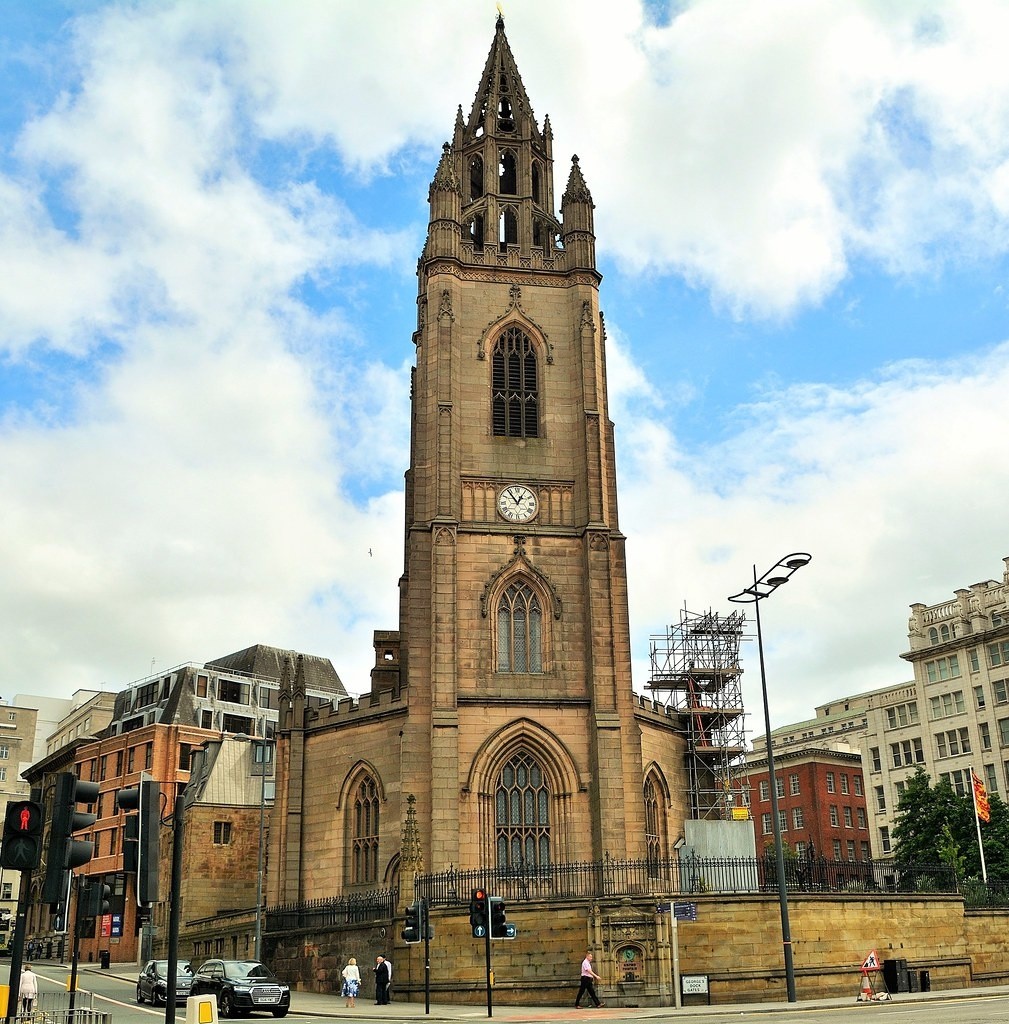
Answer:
[972, 772, 991, 823]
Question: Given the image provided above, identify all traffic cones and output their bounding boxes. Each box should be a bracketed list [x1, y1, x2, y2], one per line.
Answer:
[862, 969, 872, 999]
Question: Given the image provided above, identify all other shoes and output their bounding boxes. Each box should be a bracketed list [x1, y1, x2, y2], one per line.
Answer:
[27, 1017, 31, 1020]
[575, 1006, 583, 1009]
[387, 1001, 391, 1004]
[346, 1004, 349, 1007]
[351, 1004, 355, 1008]
[381, 1002, 387, 1005]
[597, 1003, 605, 1007]
[373, 1000, 382, 1005]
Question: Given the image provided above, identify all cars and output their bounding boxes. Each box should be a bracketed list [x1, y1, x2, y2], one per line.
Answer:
[137, 960, 195, 1006]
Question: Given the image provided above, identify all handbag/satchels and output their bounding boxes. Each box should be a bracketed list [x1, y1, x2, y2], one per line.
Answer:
[341, 978, 348, 997]
[32, 997, 37, 1006]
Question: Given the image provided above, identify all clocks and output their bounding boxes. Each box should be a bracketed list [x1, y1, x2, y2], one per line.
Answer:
[497, 484, 539, 522]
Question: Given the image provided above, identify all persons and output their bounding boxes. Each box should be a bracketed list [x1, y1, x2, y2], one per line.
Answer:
[26, 938, 35, 961]
[372, 954, 392, 1005]
[34, 940, 44, 962]
[575, 952, 606, 1009]
[19, 964, 38, 1020]
[341, 958, 361, 1007]
[44, 941, 54, 960]
[56, 940, 63, 958]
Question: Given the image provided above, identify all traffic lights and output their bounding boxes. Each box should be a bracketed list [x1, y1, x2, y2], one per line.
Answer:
[1, 801, 44, 871]
[115, 783, 137, 875]
[65, 778, 98, 872]
[492, 901, 507, 937]
[401, 907, 417, 940]
[101, 884, 110, 915]
[471, 889, 486, 924]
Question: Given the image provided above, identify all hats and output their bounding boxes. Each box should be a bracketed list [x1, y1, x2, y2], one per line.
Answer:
[25, 964, 32, 969]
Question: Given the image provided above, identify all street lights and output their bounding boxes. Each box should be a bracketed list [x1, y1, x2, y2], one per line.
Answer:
[727, 552, 812, 1004]
[231, 732, 268, 961]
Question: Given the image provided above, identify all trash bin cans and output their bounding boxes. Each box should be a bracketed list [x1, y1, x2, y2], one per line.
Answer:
[884, 958, 909, 993]
[101, 951, 110, 969]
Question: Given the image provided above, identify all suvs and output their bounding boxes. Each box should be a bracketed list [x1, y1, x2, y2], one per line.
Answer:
[189, 959, 290, 1017]
